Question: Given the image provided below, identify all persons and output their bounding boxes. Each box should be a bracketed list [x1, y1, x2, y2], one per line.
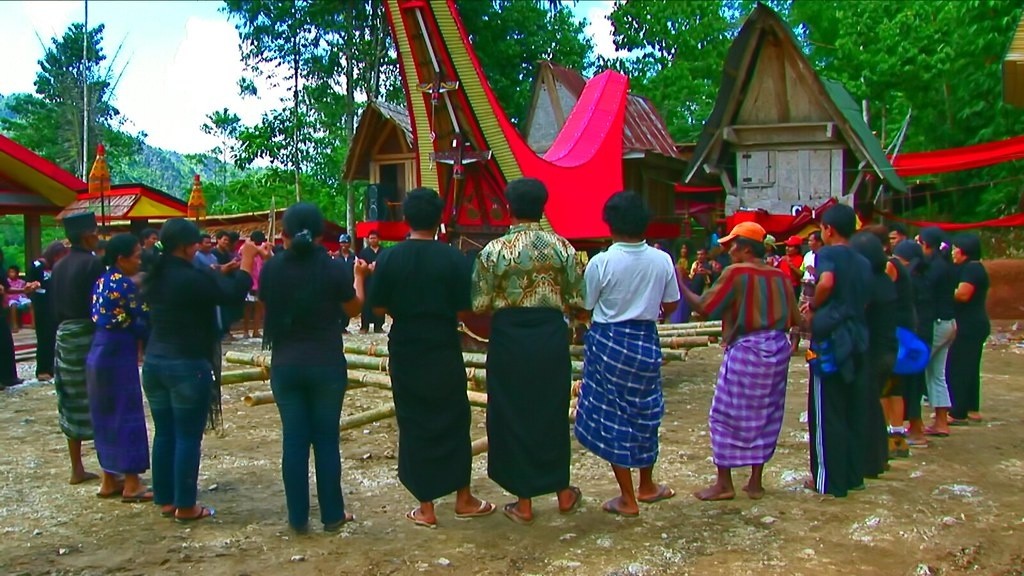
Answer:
[576, 189, 682, 518]
[366, 187, 497, 527]
[85, 233, 153, 503]
[674, 222, 803, 501]
[50, 212, 108, 484]
[140, 217, 258, 523]
[469, 176, 586, 525]
[253, 201, 367, 531]
[0, 201, 991, 498]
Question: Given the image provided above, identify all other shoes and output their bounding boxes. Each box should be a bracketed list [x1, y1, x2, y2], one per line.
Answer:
[38, 373, 52, 380]
[323, 513, 353, 533]
[0, 378, 23, 391]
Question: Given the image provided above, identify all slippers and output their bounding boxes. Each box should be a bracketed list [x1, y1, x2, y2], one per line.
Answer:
[405, 507, 437, 529]
[503, 502, 534, 526]
[174, 506, 212, 523]
[638, 484, 676, 502]
[161, 506, 177, 517]
[601, 496, 639, 518]
[804, 395, 981, 498]
[96, 481, 125, 498]
[559, 486, 582, 514]
[455, 500, 496, 518]
[122, 486, 153, 502]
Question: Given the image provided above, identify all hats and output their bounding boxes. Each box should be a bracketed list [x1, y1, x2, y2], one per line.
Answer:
[764, 234, 778, 251]
[339, 234, 351, 243]
[784, 235, 803, 246]
[62, 211, 98, 235]
[717, 222, 767, 248]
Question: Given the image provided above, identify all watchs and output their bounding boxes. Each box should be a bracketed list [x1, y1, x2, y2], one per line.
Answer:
[807, 301, 816, 312]
